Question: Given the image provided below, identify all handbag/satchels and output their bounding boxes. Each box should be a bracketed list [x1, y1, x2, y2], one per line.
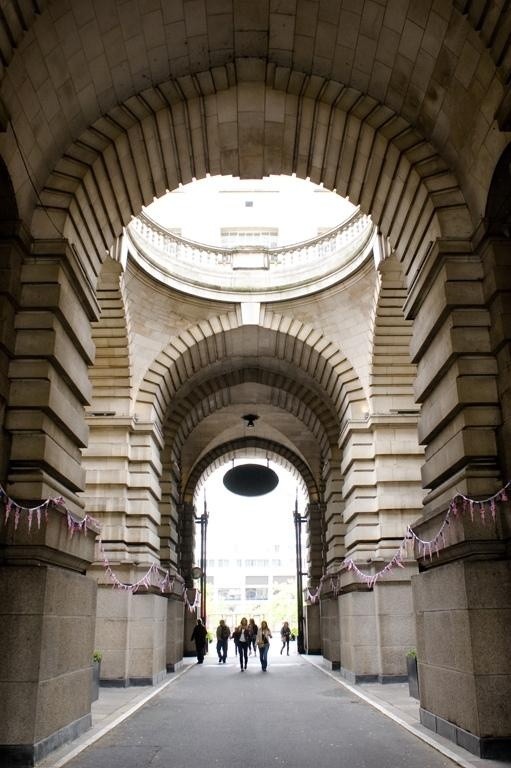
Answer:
[256, 641, 264, 648]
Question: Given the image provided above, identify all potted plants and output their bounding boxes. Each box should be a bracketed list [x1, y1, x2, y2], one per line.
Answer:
[90, 650, 102, 703]
[406, 649, 419, 700]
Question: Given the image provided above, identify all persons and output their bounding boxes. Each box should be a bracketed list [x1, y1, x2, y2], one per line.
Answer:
[232, 627, 241, 656]
[247, 618, 259, 657]
[190, 618, 208, 664]
[280, 621, 292, 656]
[235, 617, 254, 672]
[255, 620, 273, 671]
[216, 619, 231, 663]
